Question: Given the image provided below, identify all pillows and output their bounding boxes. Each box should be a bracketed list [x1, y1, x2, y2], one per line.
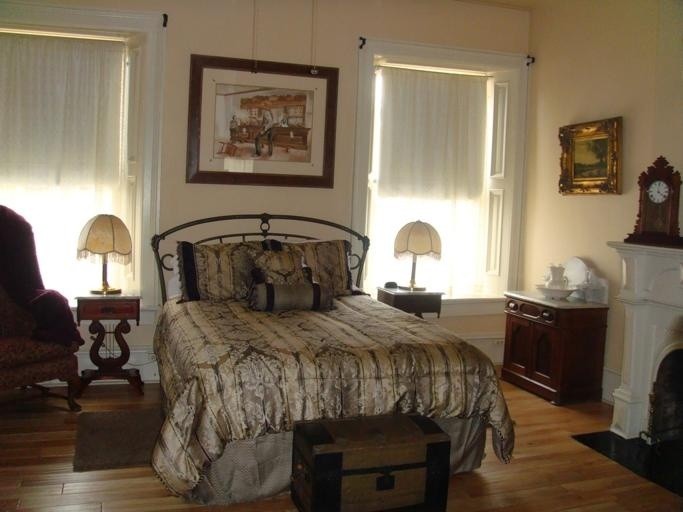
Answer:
[251, 283, 330, 312]
[260, 239, 351, 299]
[179, 239, 260, 303]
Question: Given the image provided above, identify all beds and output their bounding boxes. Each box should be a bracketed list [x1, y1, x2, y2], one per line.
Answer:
[151, 213, 501, 503]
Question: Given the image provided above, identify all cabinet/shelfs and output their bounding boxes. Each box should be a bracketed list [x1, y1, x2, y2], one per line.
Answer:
[500, 258, 610, 405]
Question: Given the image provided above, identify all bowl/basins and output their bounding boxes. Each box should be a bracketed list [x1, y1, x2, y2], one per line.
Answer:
[536, 287, 579, 300]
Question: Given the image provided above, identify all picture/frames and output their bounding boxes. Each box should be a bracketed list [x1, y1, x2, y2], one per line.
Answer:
[185, 51, 340, 189]
[556, 117, 624, 197]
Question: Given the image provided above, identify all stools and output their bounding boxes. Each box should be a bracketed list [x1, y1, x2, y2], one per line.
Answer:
[289, 414, 452, 510]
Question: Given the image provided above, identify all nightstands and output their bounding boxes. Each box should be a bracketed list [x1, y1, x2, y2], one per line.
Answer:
[74, 293, 144, 399]
[377, 285, 440, 325]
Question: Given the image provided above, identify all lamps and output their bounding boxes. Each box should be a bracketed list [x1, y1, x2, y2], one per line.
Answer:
[392, 221, 442, 290]
[76, 214, 133, 295]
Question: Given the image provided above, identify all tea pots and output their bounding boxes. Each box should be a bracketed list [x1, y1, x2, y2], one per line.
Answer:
[546, 267, 569, 290]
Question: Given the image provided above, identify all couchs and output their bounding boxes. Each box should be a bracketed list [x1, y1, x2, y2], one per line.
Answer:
[0, 205, 83, 414]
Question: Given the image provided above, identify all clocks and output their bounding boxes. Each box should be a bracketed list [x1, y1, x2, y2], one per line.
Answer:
[622, 155, 683, 249]
